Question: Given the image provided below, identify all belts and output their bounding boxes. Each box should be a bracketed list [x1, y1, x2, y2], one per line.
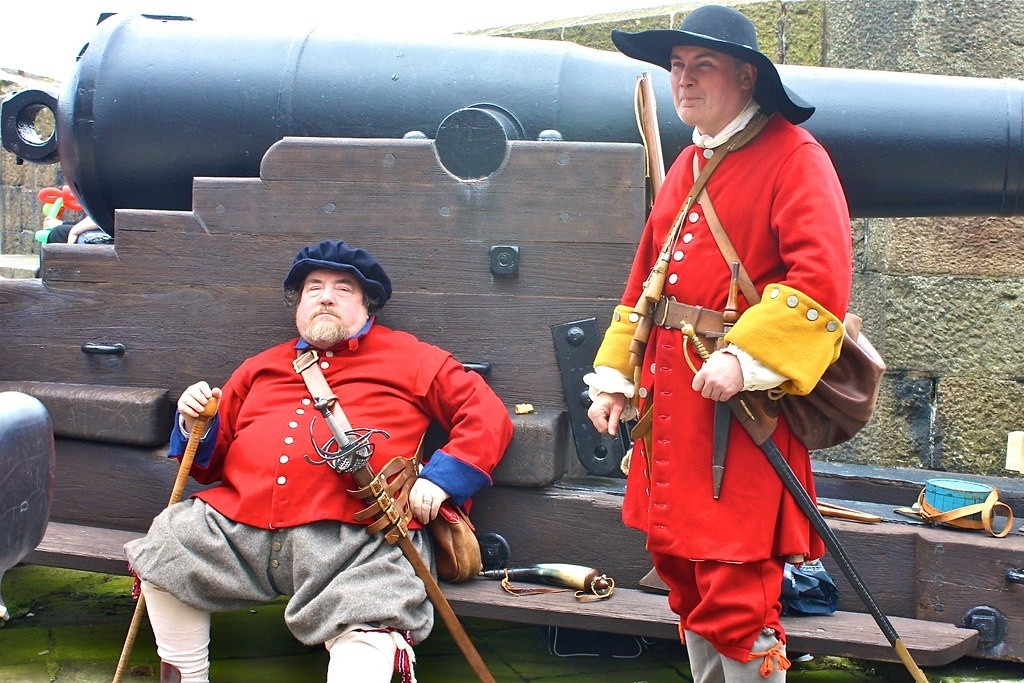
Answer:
[653, 294, 724, 338]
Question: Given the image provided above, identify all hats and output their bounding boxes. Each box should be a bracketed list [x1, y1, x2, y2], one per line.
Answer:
[611, 5, 815, 126]
[283, 240, 392, 307]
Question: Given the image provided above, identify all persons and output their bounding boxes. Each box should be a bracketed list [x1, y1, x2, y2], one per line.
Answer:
[122, 241, 514, 683]
[586, 5, 852, 683]
[33, 214, 102, 278]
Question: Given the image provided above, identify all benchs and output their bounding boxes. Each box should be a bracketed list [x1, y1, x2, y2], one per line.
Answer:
[14, 515, 980, 668]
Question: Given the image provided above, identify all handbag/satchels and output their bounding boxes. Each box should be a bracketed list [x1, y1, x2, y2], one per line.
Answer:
[428, 500, 482, 583]
[780, 314, 886, 450]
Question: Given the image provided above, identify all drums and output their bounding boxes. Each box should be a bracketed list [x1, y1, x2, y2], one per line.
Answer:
[923, 478, 994, 531]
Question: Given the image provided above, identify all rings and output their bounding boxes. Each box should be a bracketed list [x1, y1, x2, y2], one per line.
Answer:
[423, 501, 431, 506]
